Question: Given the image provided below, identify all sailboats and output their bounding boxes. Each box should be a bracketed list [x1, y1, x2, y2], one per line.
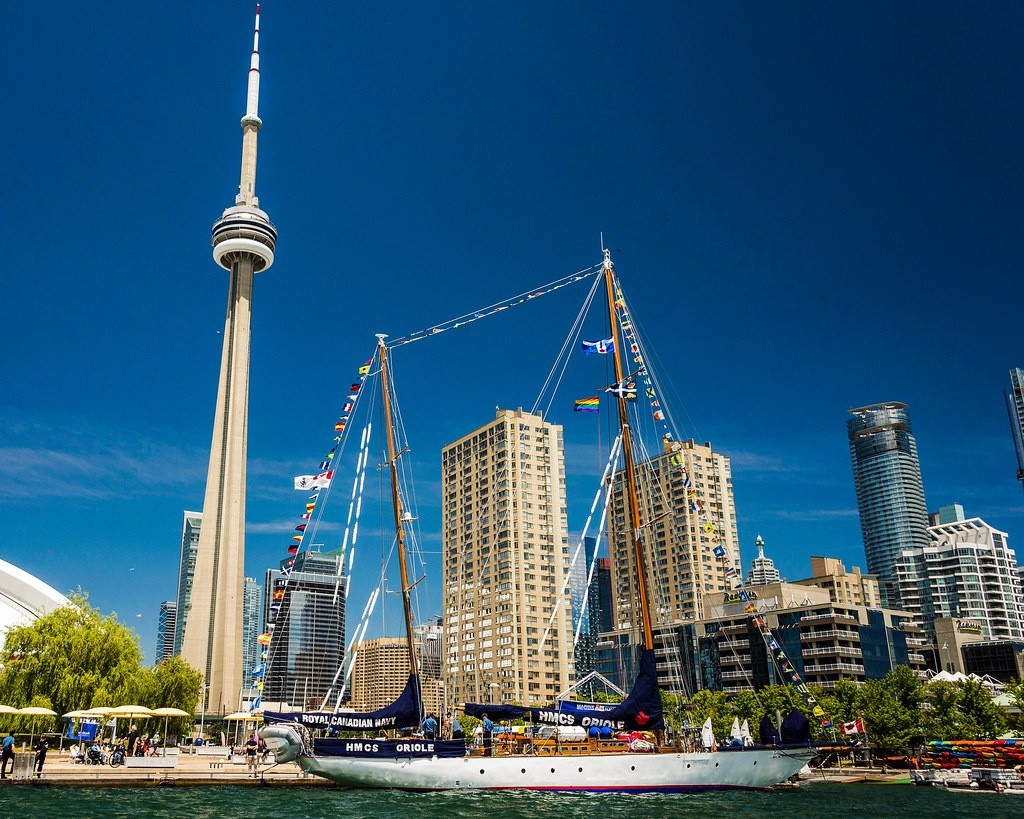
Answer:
[243, 229, 878, 795]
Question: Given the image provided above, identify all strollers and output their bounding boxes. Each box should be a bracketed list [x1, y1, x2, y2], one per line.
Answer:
[85, 746, 103, 765]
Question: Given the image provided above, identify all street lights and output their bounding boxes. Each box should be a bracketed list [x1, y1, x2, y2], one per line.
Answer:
[200, 683, 211, 739]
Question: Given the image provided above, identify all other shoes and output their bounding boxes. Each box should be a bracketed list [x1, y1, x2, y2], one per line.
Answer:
[249, 775, 252, 777]
[256, 776, 259, 777]
[38, 776, 41, 778]
[1, 777, 7, 779]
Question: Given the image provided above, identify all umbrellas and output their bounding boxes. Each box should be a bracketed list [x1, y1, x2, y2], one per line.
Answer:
[61, 705, 189, 756]
[224, 712, 263, 730]
[0, 704, 57, 746]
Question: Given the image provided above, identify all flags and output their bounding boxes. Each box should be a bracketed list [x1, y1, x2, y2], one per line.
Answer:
[615, 289, 832, 728]
[573, 397, 599, 413]
[249, 493, 319, 711]
[581, 336, 615, 354]
[293, 470, 334, 490]
[841, 719, 863, 735]
[319, 358, 373, 471]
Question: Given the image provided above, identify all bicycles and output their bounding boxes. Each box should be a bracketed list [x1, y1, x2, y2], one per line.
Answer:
[108, 742, 146, 769]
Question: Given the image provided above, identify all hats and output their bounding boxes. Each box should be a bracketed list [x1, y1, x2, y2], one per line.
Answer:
[40, 734, 46, 739]
[480, 713, 488, 717]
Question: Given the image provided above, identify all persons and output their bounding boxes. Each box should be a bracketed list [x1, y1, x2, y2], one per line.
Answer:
[729, 735, 743, 746]
[34, 734, 48, 778]
[482, 713, 493, 756]
[245, 734, 270, 777]
[917, 756, 925, 769]
[422, 713, 437, 739]
[70, 724, 159, 763]
[1, 730, 16, 779]
[446, 712, 461, 739]
